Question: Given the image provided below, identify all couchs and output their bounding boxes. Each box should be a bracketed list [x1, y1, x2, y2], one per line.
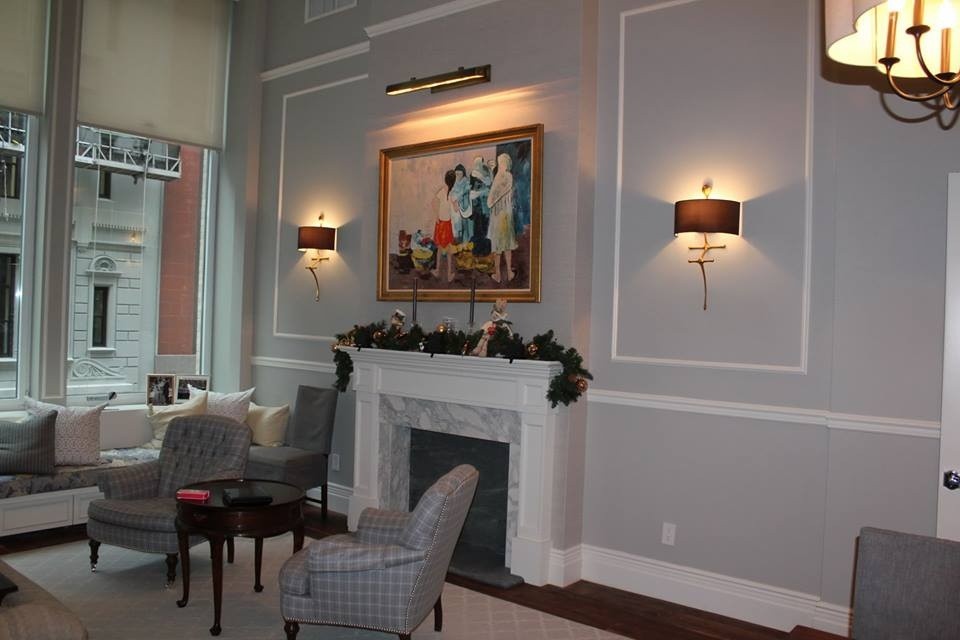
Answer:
[0, 559, 88, 640]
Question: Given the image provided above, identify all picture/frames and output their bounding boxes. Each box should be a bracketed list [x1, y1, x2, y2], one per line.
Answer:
[376, 125, 541, 304]
[175, 375, 212, 403]
[146, 373, 176, 407]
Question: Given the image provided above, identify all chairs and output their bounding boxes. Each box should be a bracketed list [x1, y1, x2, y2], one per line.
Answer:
[87, 414, 255, 590]
[244, 383, 339, 523]
[851, 525, 960, 640]
[277, 462, 480, 640]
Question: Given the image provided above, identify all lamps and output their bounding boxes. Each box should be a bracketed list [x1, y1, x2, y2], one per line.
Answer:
[673, 185, 741, 312]
[822, 0, 960, 113]
[297, 215, 336, 302]
[385, 64, 492, 96]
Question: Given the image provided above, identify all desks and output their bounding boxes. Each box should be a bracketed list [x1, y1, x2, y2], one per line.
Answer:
[173, 478, 308, 636]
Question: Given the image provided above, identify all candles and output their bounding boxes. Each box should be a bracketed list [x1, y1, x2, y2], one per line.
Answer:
[412, 277, 417, 321]
[470, 279, 476, 323]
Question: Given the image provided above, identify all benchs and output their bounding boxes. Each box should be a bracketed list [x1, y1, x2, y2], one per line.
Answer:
[0, 403, 180, 548]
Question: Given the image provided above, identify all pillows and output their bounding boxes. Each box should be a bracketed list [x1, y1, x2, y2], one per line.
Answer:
[23, 397, 106, 466]
[142, 391, 208, 450]
[246, 401, 290, 447]
[187, 383, 256, 421]
[0, 409, 58, 476]
